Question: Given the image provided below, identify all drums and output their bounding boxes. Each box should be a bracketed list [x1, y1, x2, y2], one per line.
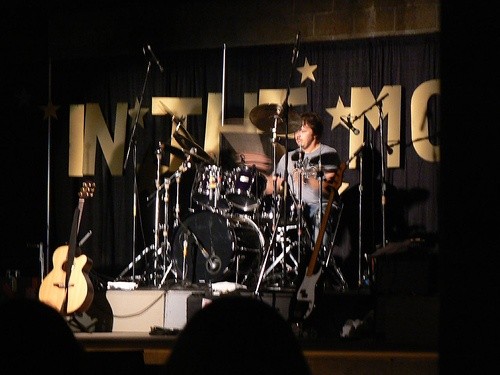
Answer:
[224, 165, 268, 210]
[235, 212, 276, 226]
[292, 166, 340, 208]
[191, 164, 231, 213]
[173, 212, 265, 284]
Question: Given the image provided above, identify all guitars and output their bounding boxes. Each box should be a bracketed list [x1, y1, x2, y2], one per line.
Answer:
[38, 181, 96, 314]
[296, 162, 346, 319]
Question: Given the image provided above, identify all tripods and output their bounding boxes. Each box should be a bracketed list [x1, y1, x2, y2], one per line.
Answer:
[123, 31, 393, 290]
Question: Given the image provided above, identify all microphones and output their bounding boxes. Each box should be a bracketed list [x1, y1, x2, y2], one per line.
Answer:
[340, 117, 360, 135]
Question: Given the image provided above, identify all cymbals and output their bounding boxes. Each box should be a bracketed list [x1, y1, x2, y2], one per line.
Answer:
[249, 103, 302, 133]
[159, 101, 194, 142]
[173, 132, 215, 162]
[164, 144, 209, 164]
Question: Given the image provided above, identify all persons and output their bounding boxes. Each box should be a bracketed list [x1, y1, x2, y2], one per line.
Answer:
[236, 110, 339, 266]
[165, 292, 313, 375]
[0, 299, 93, 375]
